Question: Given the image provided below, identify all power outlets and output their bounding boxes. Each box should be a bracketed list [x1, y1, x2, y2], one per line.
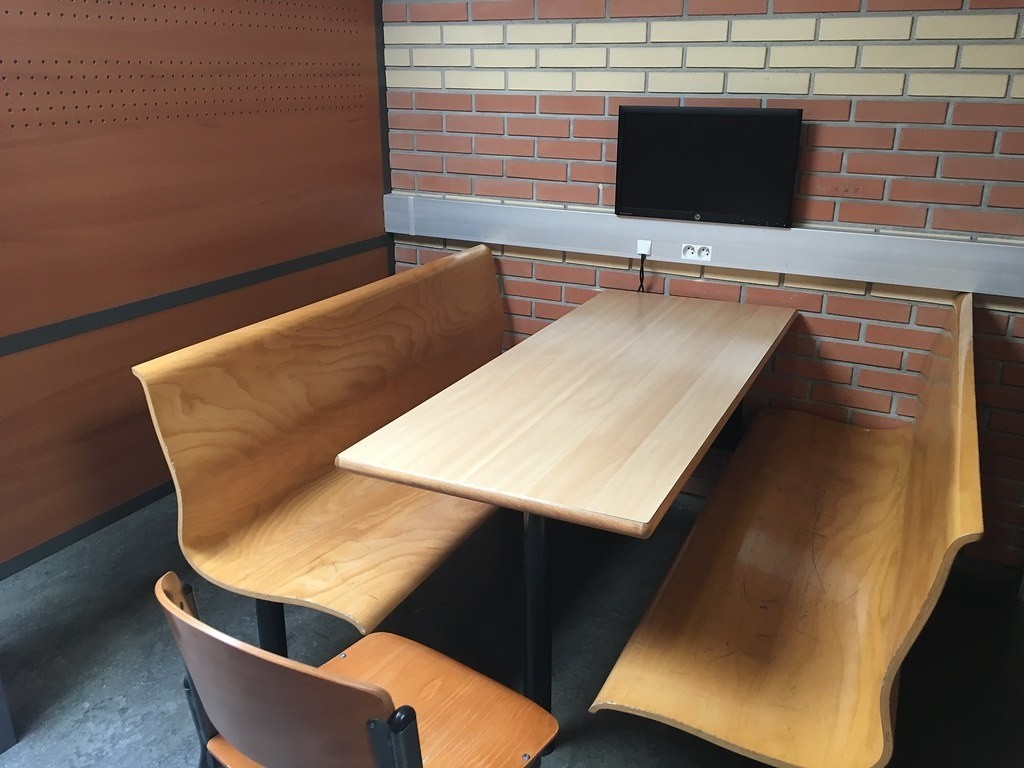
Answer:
[681, 244, 712, 262]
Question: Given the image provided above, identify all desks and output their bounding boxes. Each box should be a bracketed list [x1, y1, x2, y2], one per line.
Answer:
[335, 287, 799, 715]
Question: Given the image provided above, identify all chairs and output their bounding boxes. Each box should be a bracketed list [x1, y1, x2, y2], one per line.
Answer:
[153, 572, 560, 768]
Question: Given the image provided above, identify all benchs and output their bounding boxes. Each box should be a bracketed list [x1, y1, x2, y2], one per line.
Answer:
[588, 293, 985, 768]
[132, 245, 500, 661]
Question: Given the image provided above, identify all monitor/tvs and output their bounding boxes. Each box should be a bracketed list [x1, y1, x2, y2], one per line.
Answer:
[615, 105, 803, 228]
